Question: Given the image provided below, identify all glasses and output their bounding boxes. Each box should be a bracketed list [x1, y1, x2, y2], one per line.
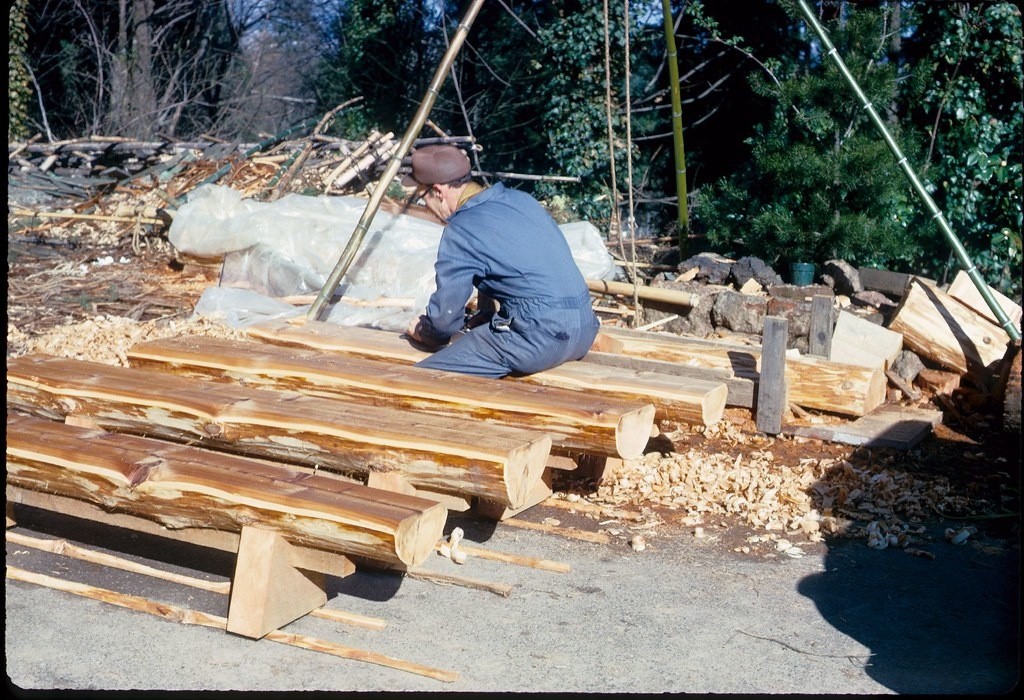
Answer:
[415, 186, 432, 207]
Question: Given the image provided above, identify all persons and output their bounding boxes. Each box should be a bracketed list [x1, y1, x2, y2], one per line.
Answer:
[401, 145, 601, 380]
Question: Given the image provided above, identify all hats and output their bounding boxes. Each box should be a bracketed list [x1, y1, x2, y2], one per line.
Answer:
[401, 145, 471, 187]
